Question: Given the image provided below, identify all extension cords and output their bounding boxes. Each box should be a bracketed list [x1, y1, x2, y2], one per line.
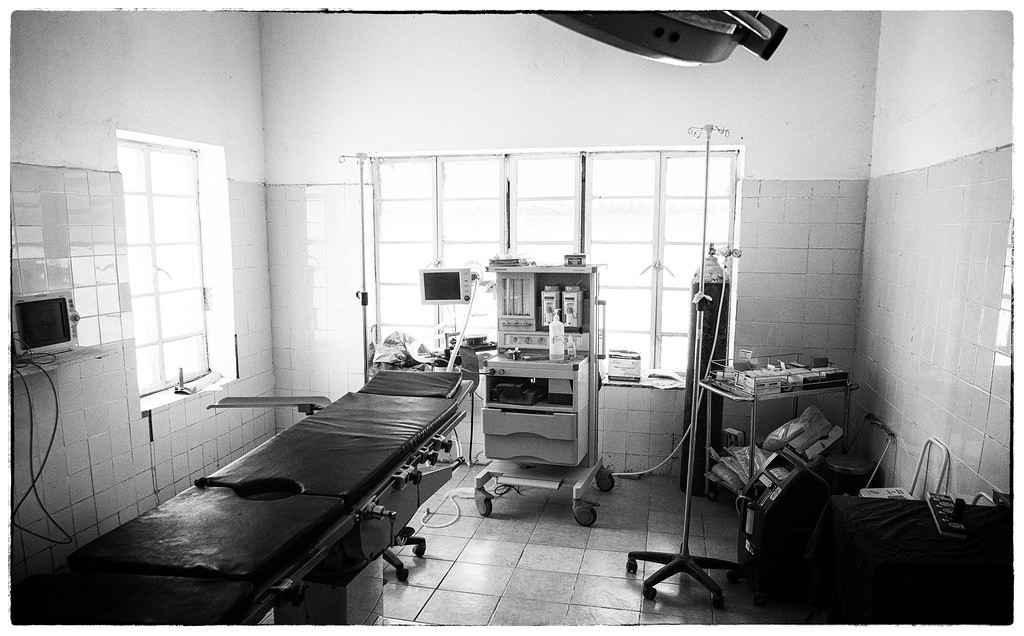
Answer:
[926, 492, 968, 540]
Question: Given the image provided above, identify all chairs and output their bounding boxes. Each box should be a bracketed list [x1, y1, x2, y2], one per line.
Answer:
[827, 411, 894, 495]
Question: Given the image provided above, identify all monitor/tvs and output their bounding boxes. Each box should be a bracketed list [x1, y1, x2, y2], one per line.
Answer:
[16, 298, 71, 350]
[424, 272, 461, 300]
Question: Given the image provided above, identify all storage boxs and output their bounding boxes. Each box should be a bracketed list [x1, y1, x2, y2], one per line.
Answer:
[742, 376, 781, 395]
[273, 554, 384, 625]
[787, 420, 845, 462]
[788, 368, 844, 388]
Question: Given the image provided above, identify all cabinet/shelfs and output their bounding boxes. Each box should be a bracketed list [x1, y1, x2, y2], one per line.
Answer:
[474, 263, 615, 527]
[699, 352, 859, 501]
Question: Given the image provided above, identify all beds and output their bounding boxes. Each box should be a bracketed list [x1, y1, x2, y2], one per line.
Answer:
[11, 372, 474, 626]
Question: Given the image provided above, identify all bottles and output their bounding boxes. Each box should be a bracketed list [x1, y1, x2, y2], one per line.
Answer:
[549, 308, 565, 361]
[567, 336, 577, 358]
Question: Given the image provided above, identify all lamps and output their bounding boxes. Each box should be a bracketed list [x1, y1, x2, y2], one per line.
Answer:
[533, 11, 788, 68]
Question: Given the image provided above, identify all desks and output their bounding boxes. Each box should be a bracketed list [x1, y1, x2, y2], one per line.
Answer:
[803, 496, 1014, 625]
